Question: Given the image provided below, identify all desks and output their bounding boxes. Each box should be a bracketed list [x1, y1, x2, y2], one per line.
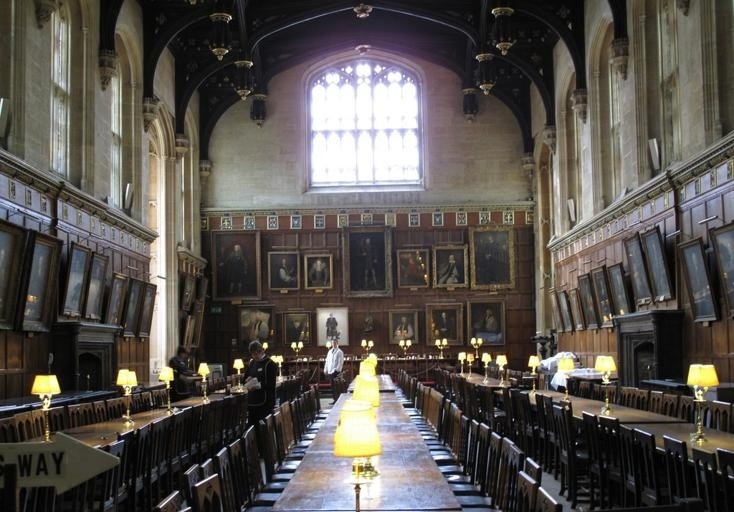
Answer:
[271, 374, 462, 512]
[281, 358, 450, 385]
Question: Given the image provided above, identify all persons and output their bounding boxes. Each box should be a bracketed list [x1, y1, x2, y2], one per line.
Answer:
[276, 255, 294, 287]
[386, 299, 507, 345]
[219, 243, 248, 296]
[436, 253, 461, 284]
[168, 346, 190, 392]
[475, 231, 510, 284]
[242, 341, 278, 458]
[323, 338, 344, 404]
[400, 250, 426, 287]
[235, 303, 341, 349]
[308, 257, 329, 286]
[357, 236, 378, 288]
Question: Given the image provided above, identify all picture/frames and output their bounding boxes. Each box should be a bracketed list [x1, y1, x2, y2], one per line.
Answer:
[548, 222, 734, 334]
[0, 218, 157, 342]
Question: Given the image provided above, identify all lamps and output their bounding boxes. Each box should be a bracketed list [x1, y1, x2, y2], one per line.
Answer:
[196, 361, 210, 400]
[209, 0, 270, 128]
[457, 350, 617, 414]
[462, 0, 518, 125]
[233, 357, 244, 387]
[353, 0, 374, 56]
